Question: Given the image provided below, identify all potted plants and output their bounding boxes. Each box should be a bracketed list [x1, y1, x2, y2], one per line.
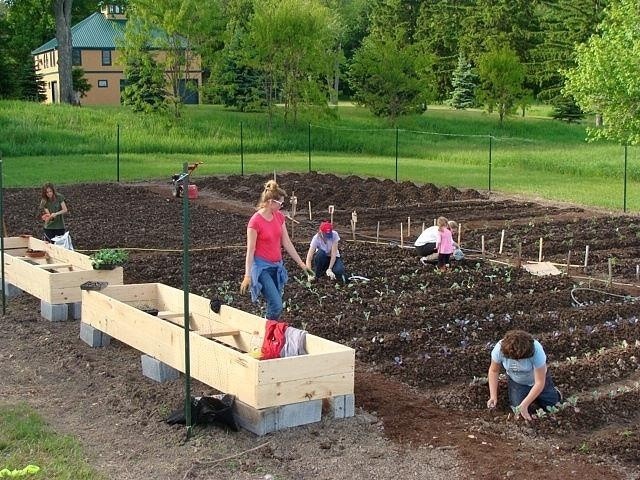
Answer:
[90, 247, 131, 270]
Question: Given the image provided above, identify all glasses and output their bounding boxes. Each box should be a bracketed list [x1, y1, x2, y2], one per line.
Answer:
[268, 199, 284, 207]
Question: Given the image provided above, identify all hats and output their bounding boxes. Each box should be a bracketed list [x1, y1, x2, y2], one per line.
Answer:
[320, 222, 333, 233]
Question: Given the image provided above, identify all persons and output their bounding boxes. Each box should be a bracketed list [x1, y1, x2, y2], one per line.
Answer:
[435, 215, 455, 274]
[486, 330, 562, 423]
[36, 182, 68, 245]
[305, 221, 348, 283]
[415, 218, 459, 265]
[238, 178, 316, 320]
